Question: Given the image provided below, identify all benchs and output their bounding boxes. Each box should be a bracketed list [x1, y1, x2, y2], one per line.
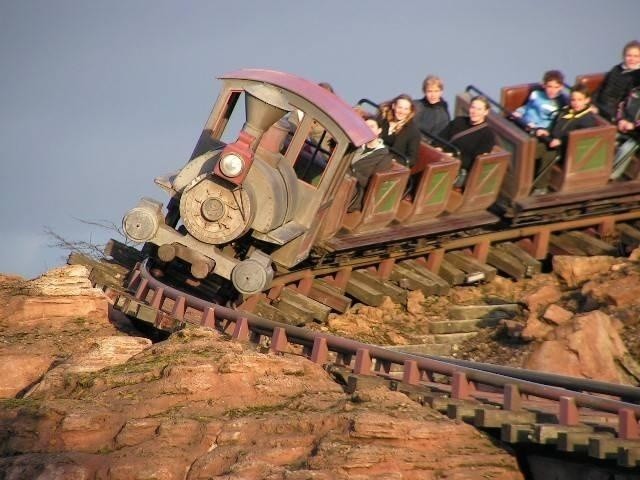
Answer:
[288, 72, 640, 233]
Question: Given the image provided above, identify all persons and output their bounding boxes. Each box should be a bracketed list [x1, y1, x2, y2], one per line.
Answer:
[410, 73, 452, 145]
[607, 84, 640, 182]
[351, 94, 421, 173]
[428, 94, 496, 192]
[345, 116, 394, 213]
[508, 67, 566, 136]
[591, 40, 640, 120]
[288, 82, 335, 183]
[531, 82, 596, 197]
